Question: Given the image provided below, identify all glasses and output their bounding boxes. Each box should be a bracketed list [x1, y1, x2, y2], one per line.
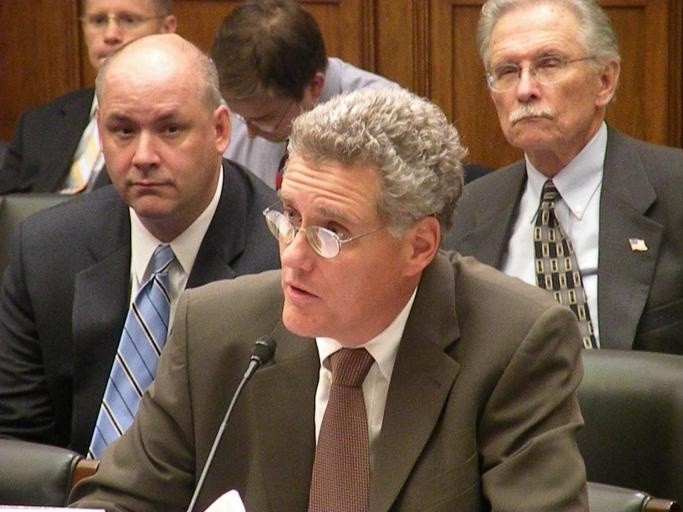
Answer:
[481, 55, 600, 86]
[78, 10, 165, 29]
[232, 99, 300, 131]
[263, 197, 385, 258]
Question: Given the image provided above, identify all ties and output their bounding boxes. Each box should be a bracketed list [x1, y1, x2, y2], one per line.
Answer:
[273, 139, 293, 192]
[60, 96, 101, 195]
[85, 244, 174, 464]
[308, 346, 374, 512]
[530, 182, 599, 351]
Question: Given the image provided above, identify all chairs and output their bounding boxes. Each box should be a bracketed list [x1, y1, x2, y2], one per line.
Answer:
[0, 437, 101, 507]
[0, 193, 79, 276]
[584, 481, 680, 512]
[583, 348, 683, 479]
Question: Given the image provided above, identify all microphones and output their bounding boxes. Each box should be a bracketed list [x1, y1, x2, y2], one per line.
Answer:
[186, 336, 277, 512]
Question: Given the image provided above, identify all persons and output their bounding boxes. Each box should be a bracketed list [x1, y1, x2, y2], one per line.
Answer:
[67, 86, 588, 512]
[439, 0, 683, 354]
[0, 0, 177, 195]
[212, 1, 402, 192]
[0, 35, 284, 461]
